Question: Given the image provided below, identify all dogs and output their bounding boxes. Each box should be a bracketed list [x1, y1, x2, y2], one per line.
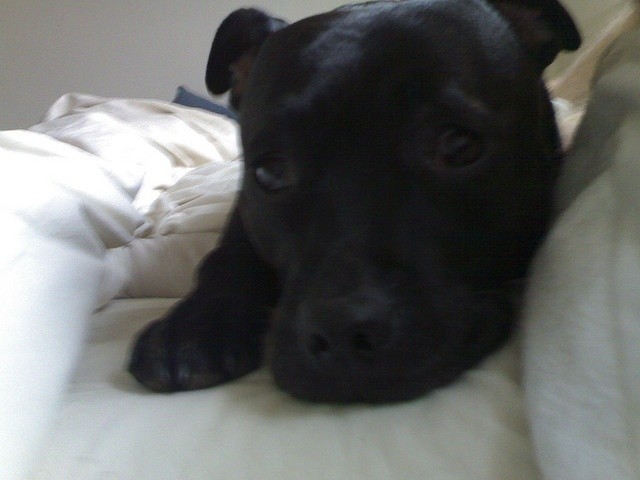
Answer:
[120, 0, 585, 411]
